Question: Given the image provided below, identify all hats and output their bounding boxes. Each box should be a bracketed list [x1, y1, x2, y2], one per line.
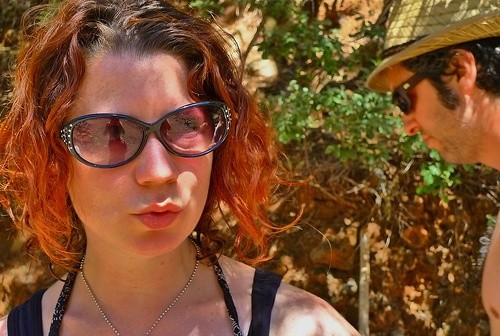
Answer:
[366, 0, 500, 94]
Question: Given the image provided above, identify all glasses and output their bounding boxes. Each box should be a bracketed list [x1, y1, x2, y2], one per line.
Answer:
[391, 71, 427, 115]
[59, 99, 232, 170]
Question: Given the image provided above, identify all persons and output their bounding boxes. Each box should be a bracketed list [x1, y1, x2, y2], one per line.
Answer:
[104, 116, 126, 165]
[367, 0, 499, 335]
[0, 0, 361, 336]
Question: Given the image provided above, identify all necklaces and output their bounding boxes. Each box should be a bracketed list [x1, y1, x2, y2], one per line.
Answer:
[79, 236, 199, 336]
